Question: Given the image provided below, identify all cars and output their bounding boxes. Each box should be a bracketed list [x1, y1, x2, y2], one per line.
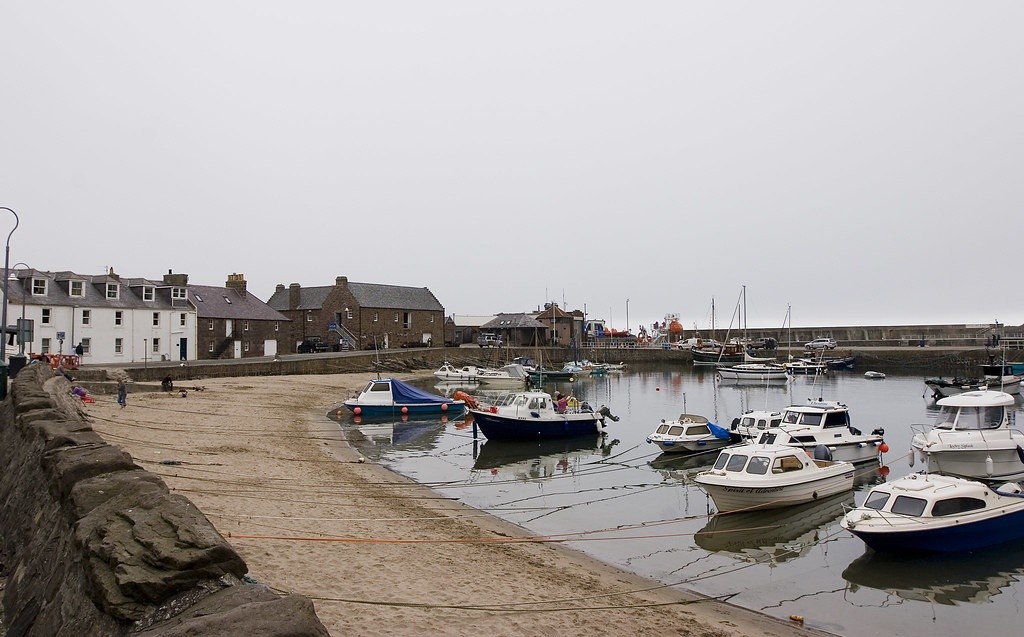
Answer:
[444, 340, 460, 348]
[401, 340, 418, 349]
[700, 339, 721, 348]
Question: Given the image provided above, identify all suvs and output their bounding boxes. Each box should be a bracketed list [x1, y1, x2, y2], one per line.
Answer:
[677, 337, 704, 351]
[477, 334, 503, 348]
[747, 337, 779, 350]
[298, 338, 329, 354]
[805, 338, 837, 350]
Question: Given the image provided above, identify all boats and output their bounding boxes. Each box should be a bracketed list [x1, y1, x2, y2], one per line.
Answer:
[646, 392, 734, 453]
[342, 334, 466, 416]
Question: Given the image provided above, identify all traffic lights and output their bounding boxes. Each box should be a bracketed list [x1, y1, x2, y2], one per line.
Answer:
[690, 284, 1024, 557]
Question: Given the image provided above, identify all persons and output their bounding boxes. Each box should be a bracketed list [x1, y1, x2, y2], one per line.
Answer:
[554, 388, 566, 414]
[69, 385, 88, 407]
[71, 342, 84, 367]
[161, 374, 173, 393]
[117, 378, 127, 409]
[581, 401, 594, 413]
[39, 352, 51, 364]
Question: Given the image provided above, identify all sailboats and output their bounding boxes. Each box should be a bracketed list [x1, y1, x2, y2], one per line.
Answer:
[469, 319, 604, 442]
[433, 300, 629, 394]
